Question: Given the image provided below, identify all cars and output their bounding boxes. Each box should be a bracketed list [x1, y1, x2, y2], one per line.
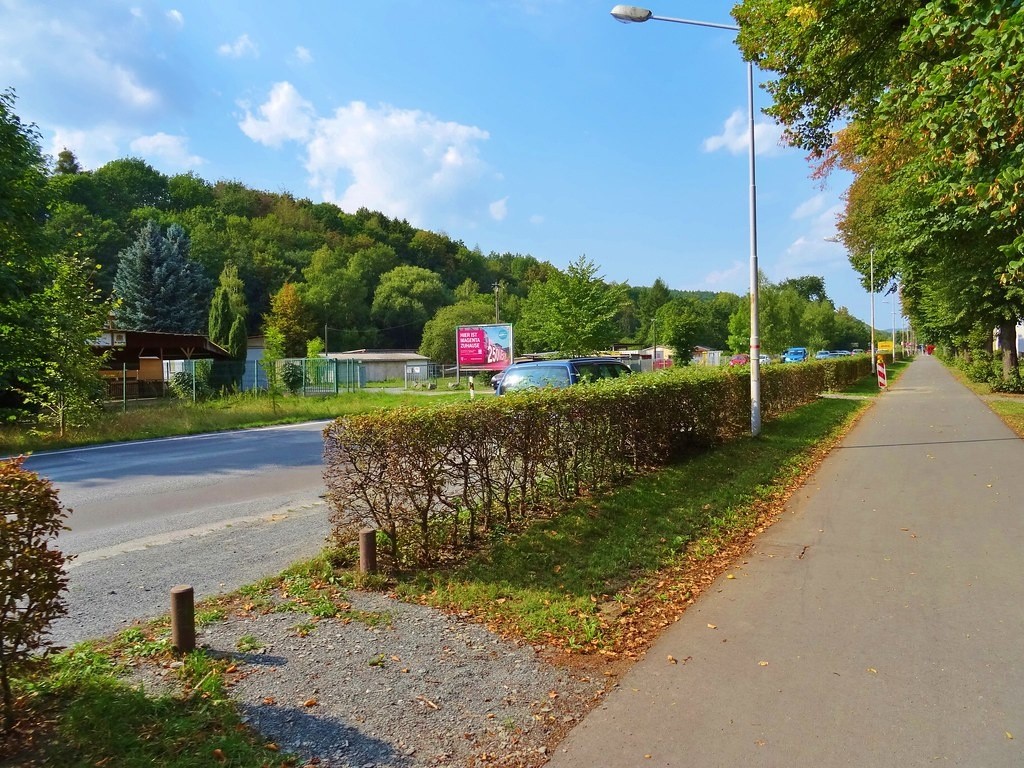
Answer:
[759, 353, 772, 367]
[730, 354, 749, 368]
[829, 349, 877, 359]
[782, 346, 809, 364]
[491, 365, 512, 390]
[816, 350, 830, 360]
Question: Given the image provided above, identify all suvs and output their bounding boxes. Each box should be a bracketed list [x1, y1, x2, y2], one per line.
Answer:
[495, 356, 634, 401]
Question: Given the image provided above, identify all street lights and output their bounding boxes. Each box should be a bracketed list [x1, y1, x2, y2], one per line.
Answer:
[612, 5, 762, 440]
[824, 236, 876, 376]
[882, 291, 896, 364]
